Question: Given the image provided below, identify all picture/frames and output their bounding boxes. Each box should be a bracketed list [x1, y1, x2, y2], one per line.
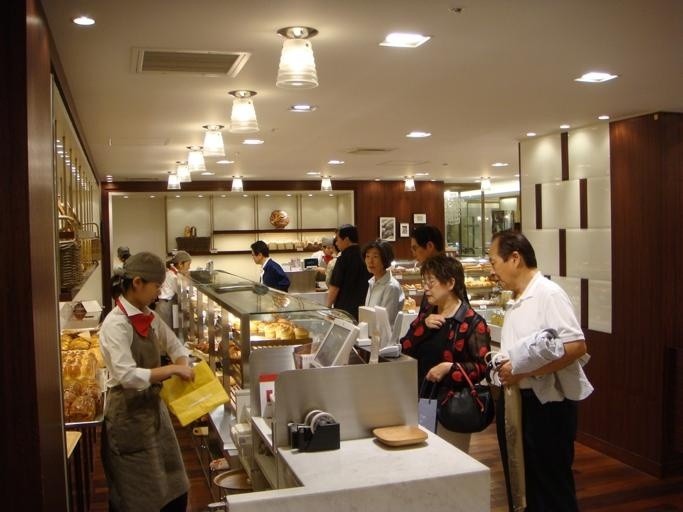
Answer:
[399, 222, 410, 238]
[379, 216, 397, 243]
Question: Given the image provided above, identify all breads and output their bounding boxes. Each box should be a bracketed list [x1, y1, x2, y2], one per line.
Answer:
[402, 297, 416, 313]
[402, 275, 496, 290]
[230, 317, 310, 342]
[60, 330, 107, 426]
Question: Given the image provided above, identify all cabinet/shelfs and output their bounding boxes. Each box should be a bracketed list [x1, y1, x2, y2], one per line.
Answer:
[388, 257, 501, 337]
[166, 227, 341, 258]
[209, 415, 492, 512]
[176, 268, 354, 422]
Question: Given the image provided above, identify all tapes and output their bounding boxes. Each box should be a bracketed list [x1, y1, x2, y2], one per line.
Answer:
[310, 412, 336, 434]
[304, 410, 324, 426]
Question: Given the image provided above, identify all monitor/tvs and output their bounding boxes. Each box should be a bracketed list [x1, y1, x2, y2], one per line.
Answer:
[311, 317, 360, 368]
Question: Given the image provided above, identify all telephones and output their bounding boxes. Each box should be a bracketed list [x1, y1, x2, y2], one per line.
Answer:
[379, 311, 403, 357]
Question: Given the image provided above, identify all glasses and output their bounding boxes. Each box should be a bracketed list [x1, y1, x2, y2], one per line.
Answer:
[419, 278, 441, 289]
[325, 246, 335, 250]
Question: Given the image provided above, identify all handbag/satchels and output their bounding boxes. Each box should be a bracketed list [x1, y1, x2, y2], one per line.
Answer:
[416, 374, 471, 455]
[438, 363, 495, 436]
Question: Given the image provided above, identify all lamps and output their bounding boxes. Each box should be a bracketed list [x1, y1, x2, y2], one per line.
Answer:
[402, 175, 417, 193]
[185, 144, 208, 174]
[320, 175, 334, 193]
[173, 159, 194, 184]
[166, 169, 182, 191]
[480, 175, 492, 193]
[200, 124, 229, 160]
[224, 88, 261, 136]
[231, 174, 245, 193]
[274, 25, 322, 91]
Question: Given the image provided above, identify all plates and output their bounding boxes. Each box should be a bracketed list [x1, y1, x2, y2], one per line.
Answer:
[372, 424, 429, 448]
[214, 466, 251, 490]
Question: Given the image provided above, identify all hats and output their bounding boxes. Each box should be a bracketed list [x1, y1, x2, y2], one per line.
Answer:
[173, 251, 192, 263]
[114, 251, 166, 284]
[118, 246, 130, 258]
[322, 236, 334, 246]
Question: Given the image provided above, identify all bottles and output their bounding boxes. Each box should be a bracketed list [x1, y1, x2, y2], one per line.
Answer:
[184, 226, 196, 237]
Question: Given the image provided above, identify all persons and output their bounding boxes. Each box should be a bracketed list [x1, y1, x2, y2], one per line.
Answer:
[401, 257, 491, 453]
[152, 252, 191, 342]
[95, 251, 196, 512]
[310, 223, 470, 333]
[112, 243, 134, 302]
[487, 230, 590, 510]
[249, 240, 290, 293]
